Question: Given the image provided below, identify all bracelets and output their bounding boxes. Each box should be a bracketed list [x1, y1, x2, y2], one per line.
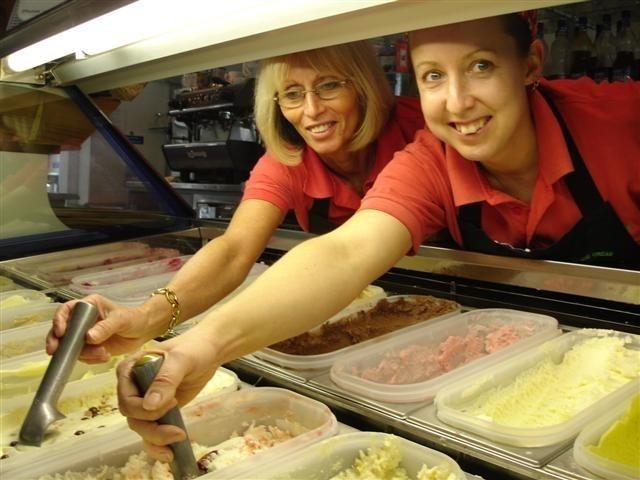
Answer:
[148, 287, 181, 340]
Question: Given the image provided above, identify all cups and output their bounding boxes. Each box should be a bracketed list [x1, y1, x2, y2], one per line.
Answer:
[225, 69, 244, 85]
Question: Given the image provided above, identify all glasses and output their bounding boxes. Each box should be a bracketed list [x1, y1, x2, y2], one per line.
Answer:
[273, 79, 353, 108]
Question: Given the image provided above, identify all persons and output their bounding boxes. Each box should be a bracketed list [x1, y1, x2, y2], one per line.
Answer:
[45, 40, 454, 365]
[116, 12, 640, 464]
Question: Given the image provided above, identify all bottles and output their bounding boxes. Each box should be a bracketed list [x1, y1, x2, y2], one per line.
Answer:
[530, 10, 639, 79]
